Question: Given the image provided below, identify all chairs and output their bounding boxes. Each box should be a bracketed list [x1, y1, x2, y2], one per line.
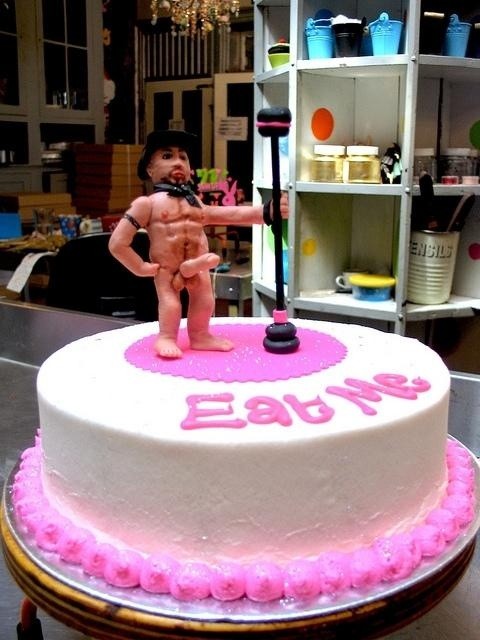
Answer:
[53, 231, 158, 323]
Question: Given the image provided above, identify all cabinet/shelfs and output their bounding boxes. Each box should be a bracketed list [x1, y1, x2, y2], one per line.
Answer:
[251, 0, 480, 332]
[1, 1, 105, 195]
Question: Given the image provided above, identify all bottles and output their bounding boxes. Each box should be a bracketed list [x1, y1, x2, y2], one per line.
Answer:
[414, 147, 479, 185]
[308, 145, 382, 185]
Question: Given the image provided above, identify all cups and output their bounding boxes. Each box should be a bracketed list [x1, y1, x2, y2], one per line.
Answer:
[336, 269, 369, 290]
[33, 207, 82, 241]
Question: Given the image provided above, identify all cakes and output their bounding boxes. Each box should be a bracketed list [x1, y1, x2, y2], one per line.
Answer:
[12, 106, 474, 600]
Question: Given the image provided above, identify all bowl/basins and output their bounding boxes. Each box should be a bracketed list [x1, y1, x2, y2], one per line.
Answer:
[268, 52, 290, 69]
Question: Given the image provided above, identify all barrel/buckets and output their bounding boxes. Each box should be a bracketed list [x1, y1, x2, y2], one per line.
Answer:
[302, 18, 335, 59]
[362, 14, 405, 55]
[444, 13, 471, 57]
[328, 24, 362, 57]
[408, 230, 462, 306]
